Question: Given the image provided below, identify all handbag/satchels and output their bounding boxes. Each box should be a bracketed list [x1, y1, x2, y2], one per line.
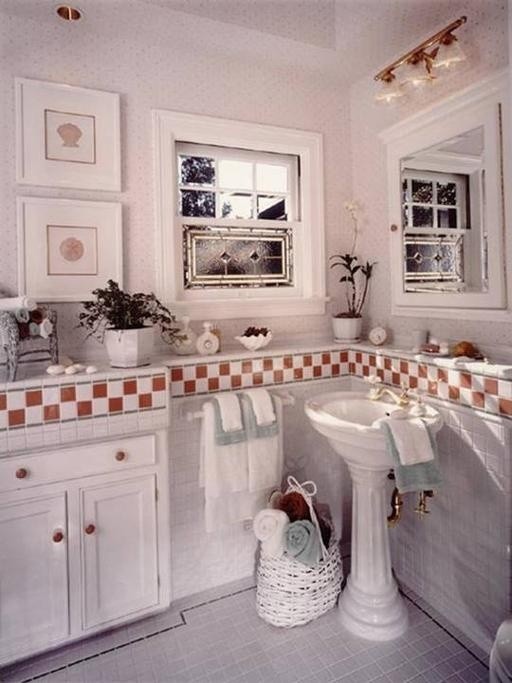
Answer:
[254, 488, 344, 629]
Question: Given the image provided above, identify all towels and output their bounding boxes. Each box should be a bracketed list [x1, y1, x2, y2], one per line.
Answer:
[372, 408, 444, 495]
[0, 297, 54, 338]
[200, 389, 336, 566]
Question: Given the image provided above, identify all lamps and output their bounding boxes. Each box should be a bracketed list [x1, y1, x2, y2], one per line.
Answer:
[372, 34, 466, 100]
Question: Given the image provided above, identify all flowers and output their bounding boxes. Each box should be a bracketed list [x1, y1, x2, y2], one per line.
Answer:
[331, 202, 376, 317]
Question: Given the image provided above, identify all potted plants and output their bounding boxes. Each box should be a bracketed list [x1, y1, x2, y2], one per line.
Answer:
[68, 280, 189, 368]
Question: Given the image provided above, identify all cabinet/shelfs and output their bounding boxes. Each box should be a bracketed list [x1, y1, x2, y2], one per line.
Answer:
[2, 429, 170, 671]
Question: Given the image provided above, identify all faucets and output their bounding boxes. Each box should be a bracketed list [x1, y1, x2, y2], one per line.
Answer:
[375, 387, 411, 406]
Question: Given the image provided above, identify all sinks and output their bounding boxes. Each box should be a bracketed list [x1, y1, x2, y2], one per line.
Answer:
[303, 391, 444, 471]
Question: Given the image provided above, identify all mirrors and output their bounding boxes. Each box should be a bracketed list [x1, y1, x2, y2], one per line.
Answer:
[155, 108, 327, 321]
[385, 102, 510, 324]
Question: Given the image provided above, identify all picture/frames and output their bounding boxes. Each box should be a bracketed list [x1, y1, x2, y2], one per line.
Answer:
[10, 76, 125, 304]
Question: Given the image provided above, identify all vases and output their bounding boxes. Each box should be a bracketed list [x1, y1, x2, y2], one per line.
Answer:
[330, 315, 364, 342]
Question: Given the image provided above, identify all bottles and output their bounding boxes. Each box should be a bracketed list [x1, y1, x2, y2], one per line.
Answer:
[210, 321, 225, 352]
[171, 315, 198, 355]
[195, 322, 219, 356]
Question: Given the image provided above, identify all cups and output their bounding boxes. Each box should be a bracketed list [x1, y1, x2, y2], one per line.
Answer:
[412, 329, 428, 353]
